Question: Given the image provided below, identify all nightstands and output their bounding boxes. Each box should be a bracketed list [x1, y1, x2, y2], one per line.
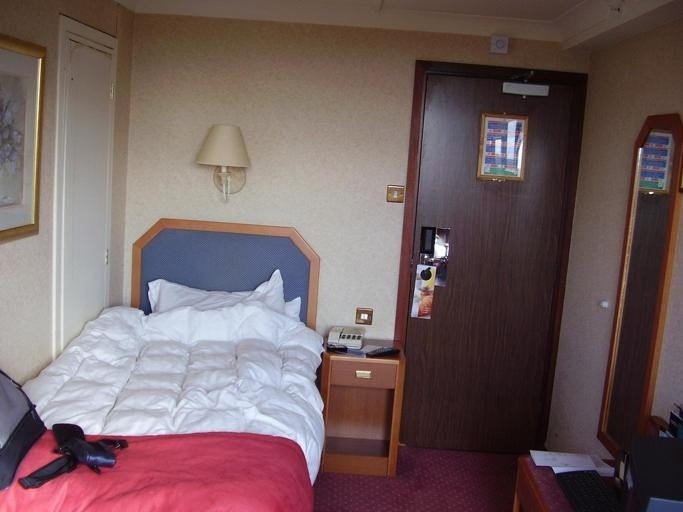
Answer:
[321, 334, 411, 478]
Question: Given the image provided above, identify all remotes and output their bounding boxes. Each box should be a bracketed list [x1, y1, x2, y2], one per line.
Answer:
[366, 347, 400, 358]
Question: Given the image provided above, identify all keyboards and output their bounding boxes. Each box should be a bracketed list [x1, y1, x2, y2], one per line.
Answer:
[556, 469, 622, 512]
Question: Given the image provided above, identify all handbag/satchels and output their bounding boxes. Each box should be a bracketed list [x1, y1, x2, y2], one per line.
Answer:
[1, 370, 47, 488]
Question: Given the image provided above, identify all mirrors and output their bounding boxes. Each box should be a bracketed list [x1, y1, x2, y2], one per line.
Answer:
[598, 108, 682, 456]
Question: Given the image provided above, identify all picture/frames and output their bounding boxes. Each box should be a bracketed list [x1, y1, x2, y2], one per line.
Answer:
[476, 110, 529, 184]
[0, 33, 49, 244]
[638, 128, 674, 196]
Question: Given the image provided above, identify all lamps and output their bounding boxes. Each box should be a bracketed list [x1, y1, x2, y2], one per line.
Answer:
[195, 117, 255, 199]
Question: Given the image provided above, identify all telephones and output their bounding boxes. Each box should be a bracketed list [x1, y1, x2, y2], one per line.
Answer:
[327, 326, 365, 350]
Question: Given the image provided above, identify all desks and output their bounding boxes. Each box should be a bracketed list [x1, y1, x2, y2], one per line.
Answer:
[511, 450, 681, 512]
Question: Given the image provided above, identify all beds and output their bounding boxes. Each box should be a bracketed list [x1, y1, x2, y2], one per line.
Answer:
[0, 215, 329, 512]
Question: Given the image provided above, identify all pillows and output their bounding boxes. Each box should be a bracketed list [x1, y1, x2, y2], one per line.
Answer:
[281, 294, 304, 326]
[146, 265, 287, 325]
